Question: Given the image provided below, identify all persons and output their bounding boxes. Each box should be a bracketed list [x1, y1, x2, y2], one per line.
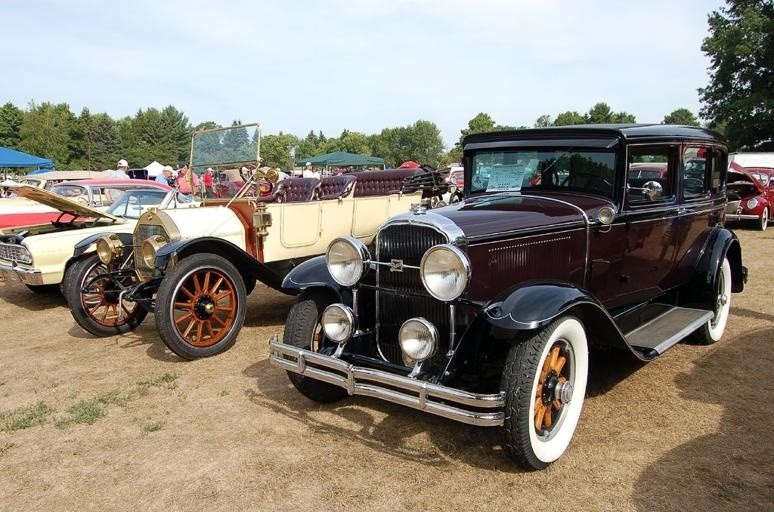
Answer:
[303, 162, 354, 179]
[450, 171, 458, 185]
[155, 162, 215, 203]
[0, 169, 61, 198]
[478, 162, 487, 177]
[104, 159, 131, 205]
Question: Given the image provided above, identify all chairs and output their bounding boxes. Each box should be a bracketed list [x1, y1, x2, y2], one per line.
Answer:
[253, 168, 358, 204]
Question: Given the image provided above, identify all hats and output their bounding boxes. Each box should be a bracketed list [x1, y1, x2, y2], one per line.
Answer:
[207, 168, 213, 171]
[118, 159, 130, 168]
[179, 161, 186, 168]
[163, 166, 174, 174]
[306, 162, 312, 166]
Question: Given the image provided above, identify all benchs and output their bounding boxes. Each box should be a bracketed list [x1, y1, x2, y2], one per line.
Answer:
[341, 169, 425, 197]
[626, 178, 666, 197]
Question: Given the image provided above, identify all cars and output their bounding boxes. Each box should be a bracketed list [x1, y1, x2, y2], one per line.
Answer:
[0, 169, 201, 294]
[60, 122, 426, 360]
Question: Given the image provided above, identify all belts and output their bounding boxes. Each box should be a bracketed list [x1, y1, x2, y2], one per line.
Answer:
[181, 192, 192, 195]
